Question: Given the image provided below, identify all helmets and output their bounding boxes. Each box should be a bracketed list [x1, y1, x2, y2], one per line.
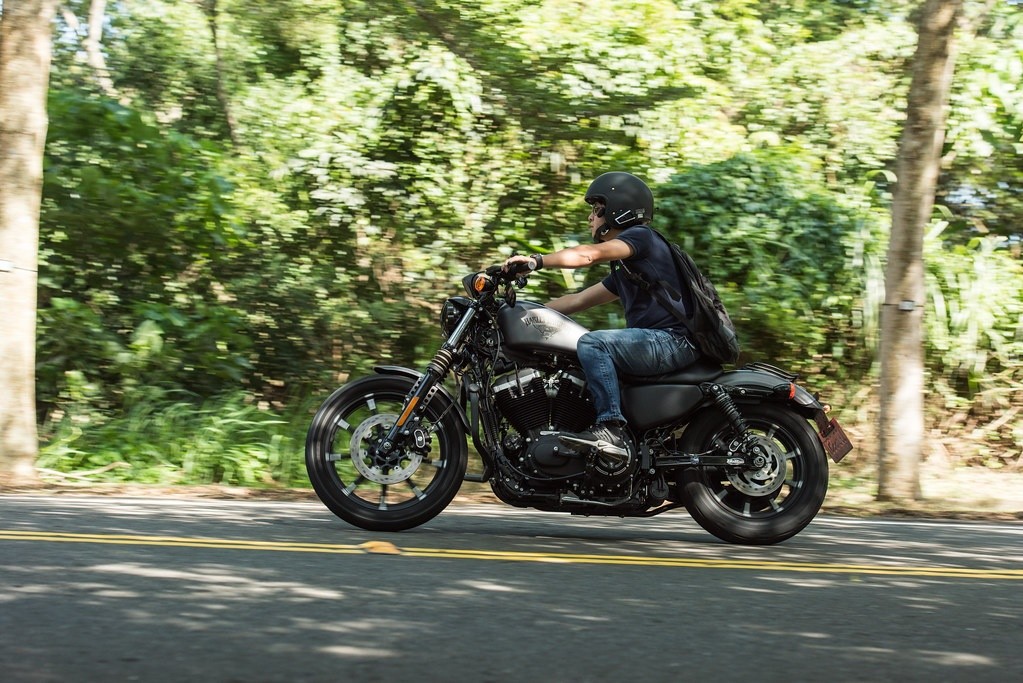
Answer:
[584, 172, 654, 228]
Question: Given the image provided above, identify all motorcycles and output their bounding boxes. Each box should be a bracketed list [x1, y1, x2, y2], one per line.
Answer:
[304, 256, 853, 546]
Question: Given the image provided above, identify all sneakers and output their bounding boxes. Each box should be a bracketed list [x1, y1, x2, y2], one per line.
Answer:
[558, 423, 628, 462]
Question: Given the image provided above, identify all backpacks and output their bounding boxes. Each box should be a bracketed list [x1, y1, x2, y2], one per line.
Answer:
[620, 228, 739, 363]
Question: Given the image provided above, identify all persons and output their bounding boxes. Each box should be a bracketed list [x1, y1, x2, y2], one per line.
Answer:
[501, 171, 705, 457]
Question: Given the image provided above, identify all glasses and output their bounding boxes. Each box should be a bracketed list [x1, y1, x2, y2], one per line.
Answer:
[592, 204, 605, 214]
